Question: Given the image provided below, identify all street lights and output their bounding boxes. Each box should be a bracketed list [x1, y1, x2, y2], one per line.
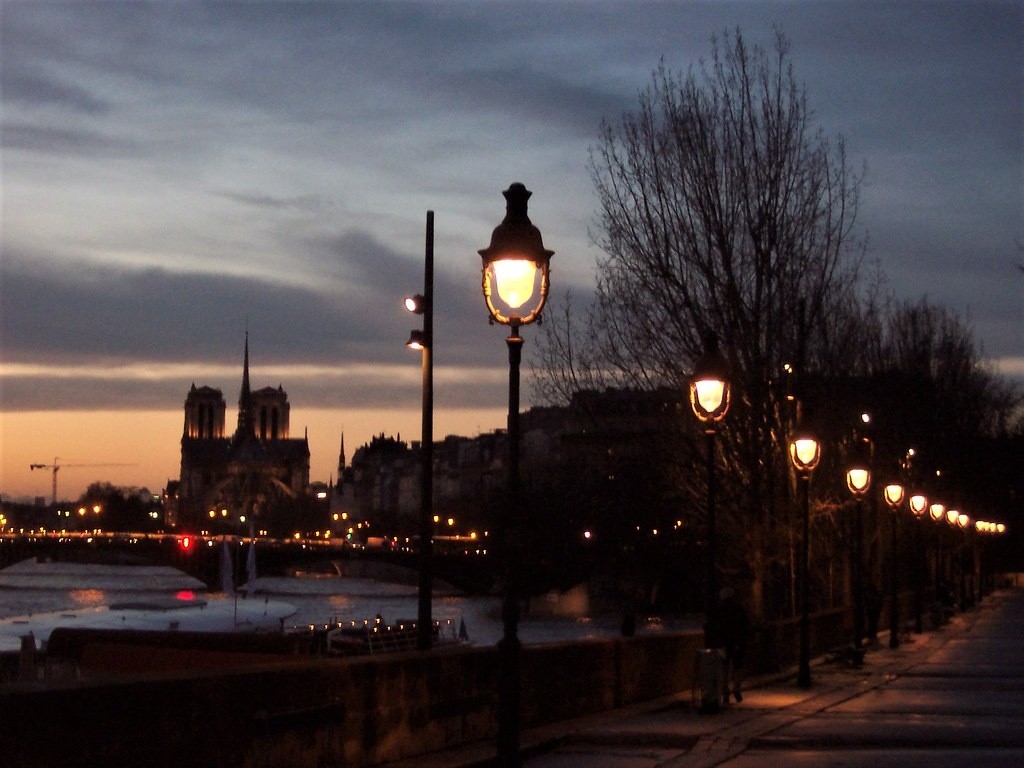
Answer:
[909, 488, 929, 634]
[784, 410, 826, 693]
[883, 473, 907, 649]
[841, 445, 877, 667]
[688, 333, 742, 708]
[930, 501, 1006, 627]
[475, 180, 556, 768]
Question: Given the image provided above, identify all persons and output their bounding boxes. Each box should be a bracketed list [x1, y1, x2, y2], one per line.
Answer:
[704, 588, 745, 702]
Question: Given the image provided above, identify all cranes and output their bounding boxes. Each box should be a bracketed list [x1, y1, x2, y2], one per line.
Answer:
[28, 457, 140, 502]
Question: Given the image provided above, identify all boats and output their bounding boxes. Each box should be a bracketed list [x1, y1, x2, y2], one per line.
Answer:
[2, 596, 301, 656]
[2, 611, 476, 707]
